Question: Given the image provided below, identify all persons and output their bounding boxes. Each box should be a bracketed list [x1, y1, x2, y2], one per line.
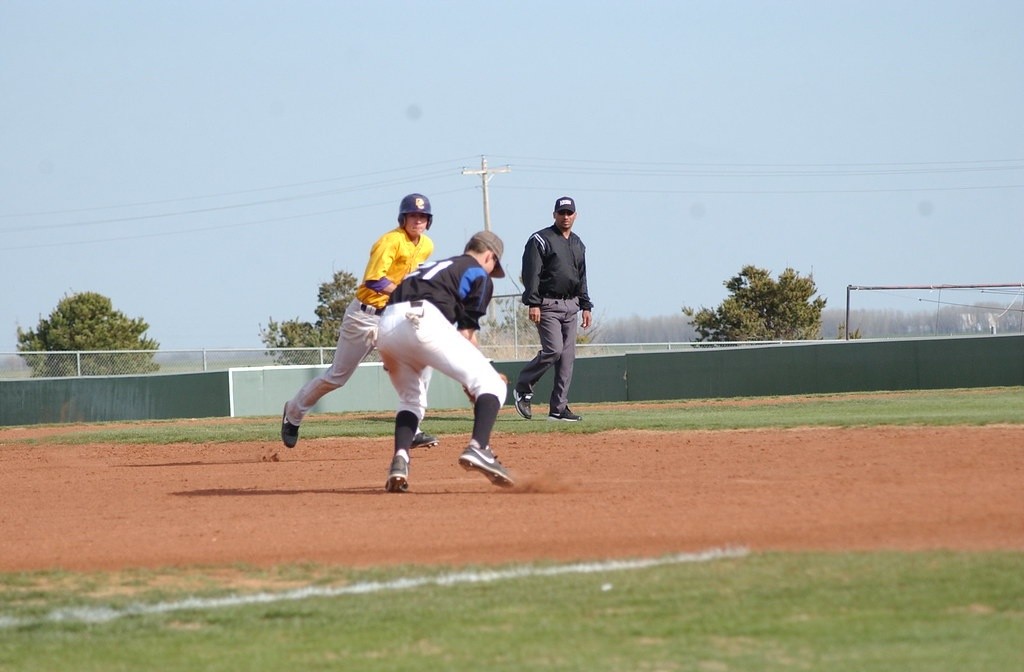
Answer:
[377, 231, 513, 491]
[514, 197, 595, 422]
[281, 193, 440, 449]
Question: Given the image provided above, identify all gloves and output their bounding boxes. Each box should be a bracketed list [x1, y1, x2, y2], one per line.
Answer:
[405, 312, 420, 328]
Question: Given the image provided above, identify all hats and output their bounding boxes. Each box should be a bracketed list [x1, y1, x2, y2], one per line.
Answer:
[472, 231, 505, 278]
[554, 197, 576, 213]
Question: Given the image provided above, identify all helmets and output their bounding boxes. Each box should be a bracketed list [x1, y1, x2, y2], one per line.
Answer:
[398, 194, 433, 229]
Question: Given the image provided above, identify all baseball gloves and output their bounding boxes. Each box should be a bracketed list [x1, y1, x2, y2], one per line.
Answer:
[462, 371, 508, 407]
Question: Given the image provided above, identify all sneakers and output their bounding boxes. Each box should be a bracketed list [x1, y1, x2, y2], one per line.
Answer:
[385, 456, 409, 490]
[410, 433, 439, 449]
[548, 405, 581, 422]
[513, 388, 534, 419]
[459, 444, 513, 487]
[281, 401, 300, 448]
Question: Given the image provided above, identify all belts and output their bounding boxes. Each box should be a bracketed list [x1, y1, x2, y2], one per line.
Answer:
[411, 301, 423, 307]
[541, 291, 575, 299]
[361, 304, 382, 316]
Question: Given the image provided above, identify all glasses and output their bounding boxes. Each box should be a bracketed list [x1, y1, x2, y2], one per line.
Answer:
[558, 210, 573, 216]
